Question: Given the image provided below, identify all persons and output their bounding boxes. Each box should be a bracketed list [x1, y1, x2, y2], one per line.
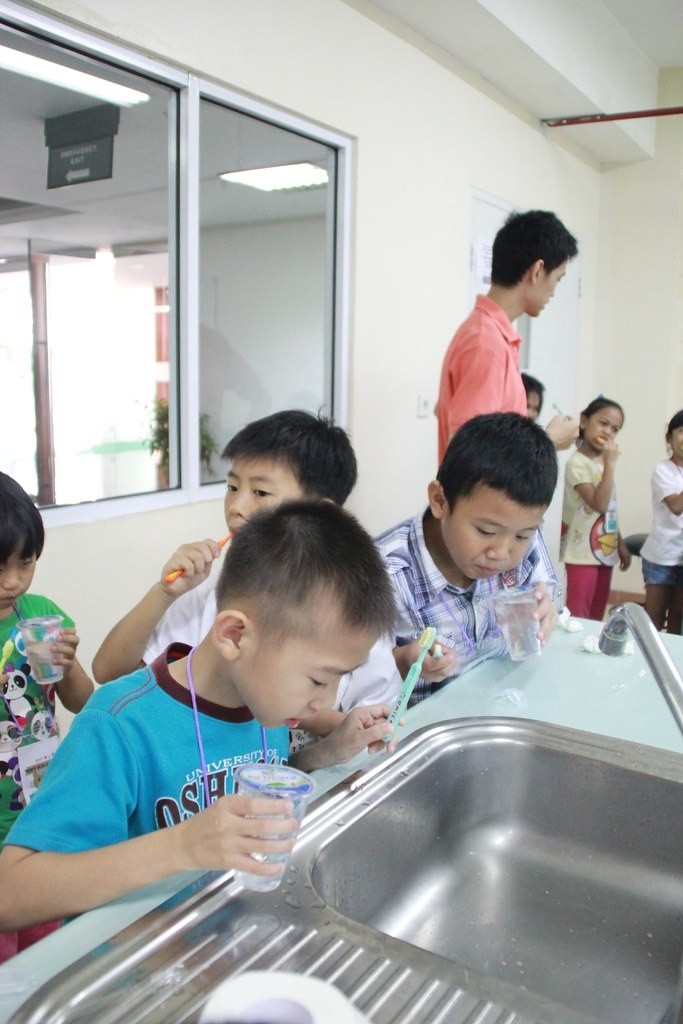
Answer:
[374, 412, 563, 708]
[92, 410, 408, 736]
[521, 373, 544, 421]
[436, 210, 578, 472]
[0, 501, 407, 932]
[640, 409, 683, 635]
[0, 471, 94, 957]
[559, 399, 632, 621]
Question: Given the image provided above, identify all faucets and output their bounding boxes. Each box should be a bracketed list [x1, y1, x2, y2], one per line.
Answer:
[599, 601, 682, 725]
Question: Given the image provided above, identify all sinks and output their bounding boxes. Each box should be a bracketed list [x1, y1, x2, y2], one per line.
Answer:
[306, 717, 681, 1020]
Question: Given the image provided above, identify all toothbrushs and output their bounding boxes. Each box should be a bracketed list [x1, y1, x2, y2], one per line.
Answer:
[595, 437, 606, 443]
[3, 639, 14, 668]
[167, 532, 232, 582]
[381, 627, 445, 744]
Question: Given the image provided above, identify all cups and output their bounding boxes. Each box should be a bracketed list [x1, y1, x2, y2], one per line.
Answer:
[15, 615, 65, 685]
[233, 762, 317, 892]
[483, 583, 543, 662]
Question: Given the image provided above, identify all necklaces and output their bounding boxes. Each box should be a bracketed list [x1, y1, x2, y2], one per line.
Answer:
[439, 578, 499, 651]
[187, 645, 267, 806]
